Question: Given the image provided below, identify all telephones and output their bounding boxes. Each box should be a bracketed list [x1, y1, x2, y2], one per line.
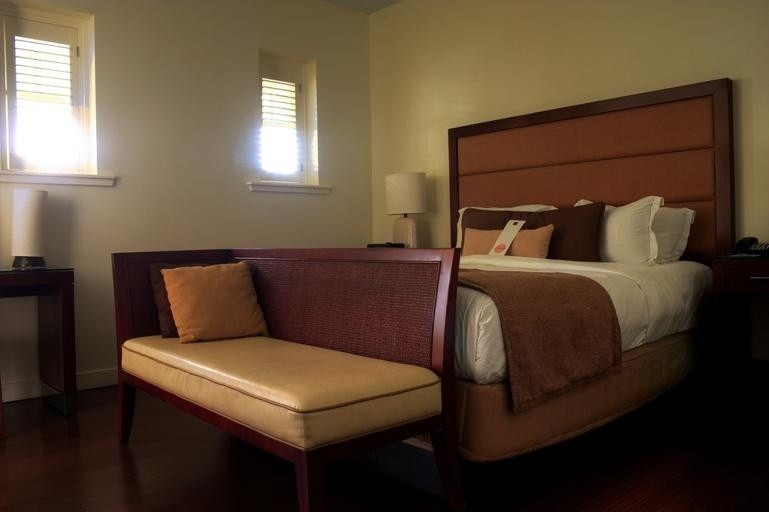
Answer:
[735, 237, 769, 253]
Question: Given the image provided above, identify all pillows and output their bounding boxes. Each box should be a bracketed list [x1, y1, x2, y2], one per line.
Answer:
[160, 257, 270, 343]
[143, 248, 236, 338]
[454, 194, 697, 264]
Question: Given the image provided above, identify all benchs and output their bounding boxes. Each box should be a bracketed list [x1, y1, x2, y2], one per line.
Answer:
[111, 243, 469, 511]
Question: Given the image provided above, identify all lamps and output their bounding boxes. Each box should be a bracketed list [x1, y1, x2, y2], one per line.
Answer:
[9, 184, 49, 272]
[382, 169, 427, 248]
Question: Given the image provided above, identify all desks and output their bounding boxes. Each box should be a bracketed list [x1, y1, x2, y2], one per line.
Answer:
[712, 251, 768, 400]
[0, 262, 84, 441]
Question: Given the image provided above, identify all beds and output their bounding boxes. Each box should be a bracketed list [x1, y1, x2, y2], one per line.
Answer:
[397, 77, 737, 499]
